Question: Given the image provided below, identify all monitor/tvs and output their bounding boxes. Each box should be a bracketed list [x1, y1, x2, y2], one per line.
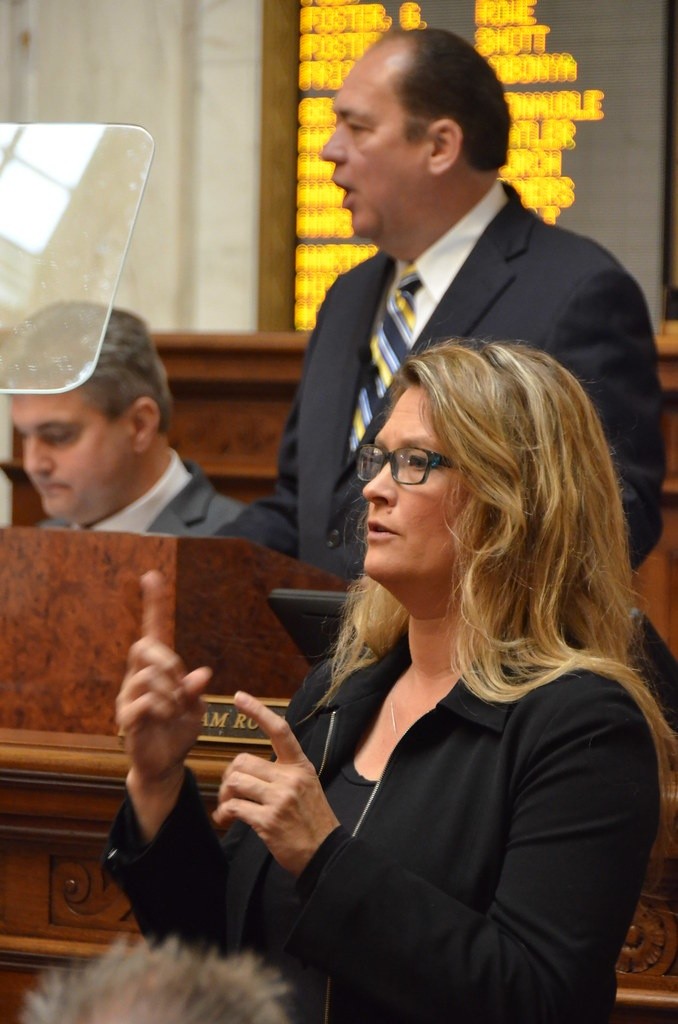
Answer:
[629, 608, 678, 730]
[264, 589, 351, 668]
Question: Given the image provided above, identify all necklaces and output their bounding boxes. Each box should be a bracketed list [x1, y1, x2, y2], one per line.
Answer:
[101, 339, 663, 1024]
[390, 679, 400, 742]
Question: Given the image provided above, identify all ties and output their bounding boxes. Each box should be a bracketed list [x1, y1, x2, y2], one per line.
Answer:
[346, 264, 423, 459]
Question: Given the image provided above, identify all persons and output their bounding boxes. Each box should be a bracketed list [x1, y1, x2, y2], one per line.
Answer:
[213, 30, 664, 581]
[0, 301, 251, 538]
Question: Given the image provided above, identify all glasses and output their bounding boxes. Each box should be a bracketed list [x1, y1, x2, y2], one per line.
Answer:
[356, 444, 461, 486]
